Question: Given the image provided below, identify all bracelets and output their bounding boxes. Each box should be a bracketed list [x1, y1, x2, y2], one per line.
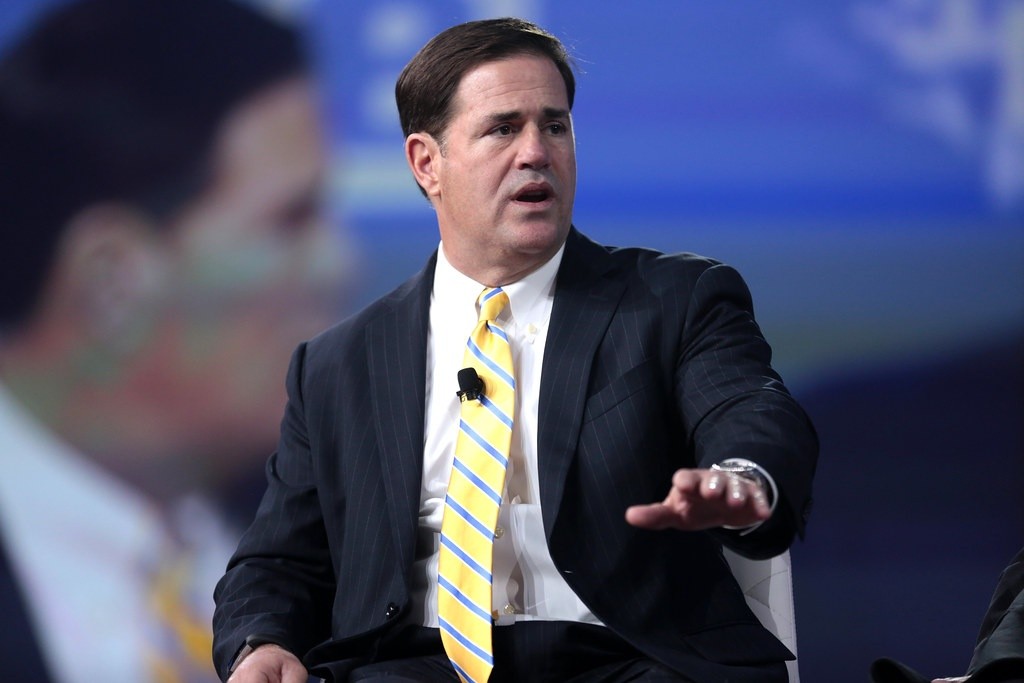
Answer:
[228, 634, 294, 678]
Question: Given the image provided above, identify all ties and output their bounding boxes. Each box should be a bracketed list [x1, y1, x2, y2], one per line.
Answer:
[437, 287, 515, 683]
[146, 549, 223, 683]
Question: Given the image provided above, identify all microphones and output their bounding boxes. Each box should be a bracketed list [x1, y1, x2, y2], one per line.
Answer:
[457, 367, 480, 400]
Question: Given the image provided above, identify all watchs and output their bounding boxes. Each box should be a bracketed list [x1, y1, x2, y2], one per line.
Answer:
[706, 461, 771, 500]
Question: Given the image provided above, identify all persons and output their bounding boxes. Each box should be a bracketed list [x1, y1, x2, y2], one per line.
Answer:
[0, 0, 369, 683]
[211, 18, 820, 683]
